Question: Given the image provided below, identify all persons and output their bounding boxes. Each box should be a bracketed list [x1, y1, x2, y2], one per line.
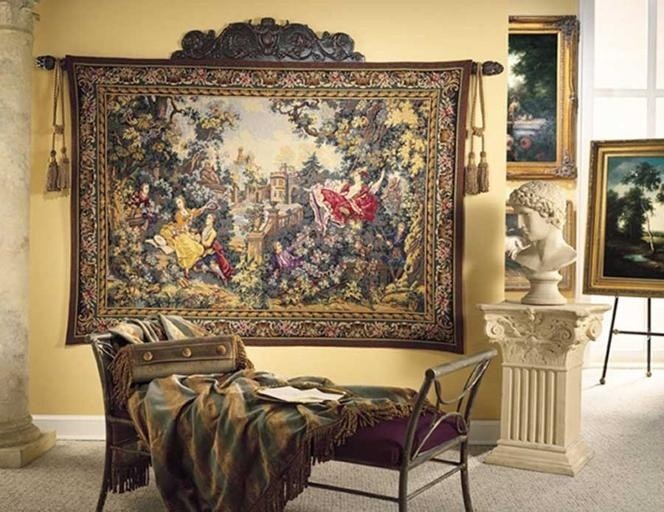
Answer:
[161, 195, 209, 280]
[507, 181, 577, 271]
[508, 96, 521, 116]
[194, 213, 227, 288]
[130, 182, 153, 215]
[302, 170, 385, 237]
[273, 240, 305, 272]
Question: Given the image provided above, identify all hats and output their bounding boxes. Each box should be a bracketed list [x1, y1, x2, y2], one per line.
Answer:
[206, 200, 222, 213]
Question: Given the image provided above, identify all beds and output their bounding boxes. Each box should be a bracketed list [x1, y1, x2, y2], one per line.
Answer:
[90, 330, 498, 512]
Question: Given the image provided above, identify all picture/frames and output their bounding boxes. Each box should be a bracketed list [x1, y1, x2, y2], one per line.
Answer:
[505, 200, 574, 291]
[507, 14, 577, 180]
[583, 138, 664, 298]
